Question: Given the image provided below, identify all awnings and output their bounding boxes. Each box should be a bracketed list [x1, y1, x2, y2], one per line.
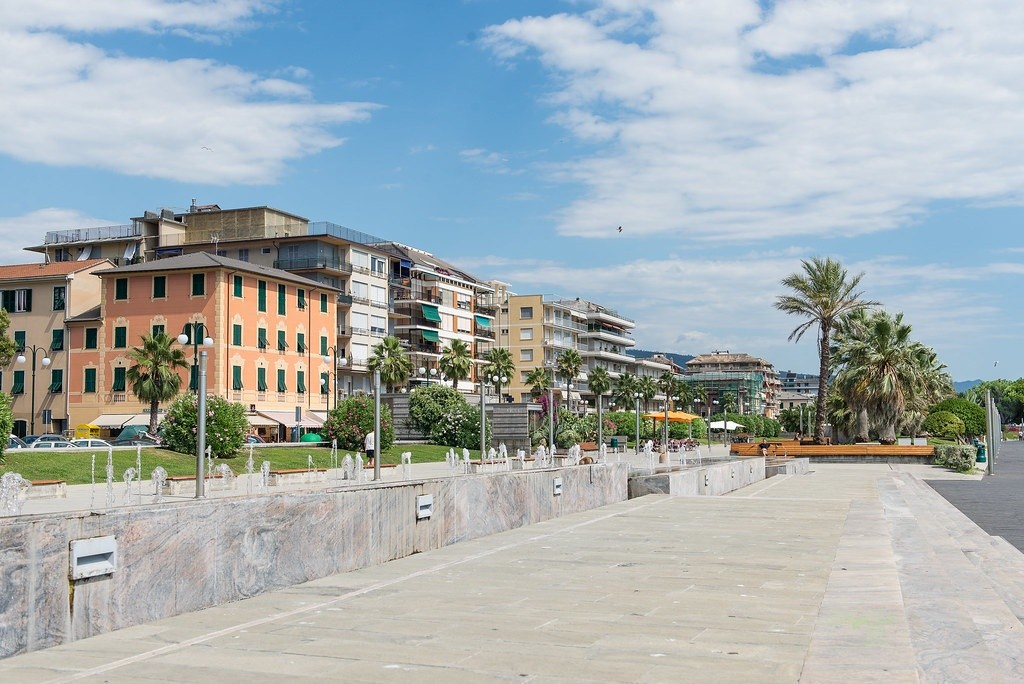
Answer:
[89, 415, 133, 429]
[476, 317, 491, 327]
[422, 331, 443, 343]
[244, 415, 280, 443]
[422, 305, 442, 322]
[123, 414, 166, 428]
[259, 411, 324, 434]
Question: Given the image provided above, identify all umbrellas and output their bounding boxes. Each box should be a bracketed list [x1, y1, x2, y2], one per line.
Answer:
[643, 411, 702, 439]
[710, 420, 746, 439]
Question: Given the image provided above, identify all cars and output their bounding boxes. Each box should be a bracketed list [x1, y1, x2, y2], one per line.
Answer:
[245, 434, 265, 443]
[10, 435, 159, 448]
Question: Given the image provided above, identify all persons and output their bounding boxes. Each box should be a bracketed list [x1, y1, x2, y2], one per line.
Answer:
[1018, 430, 1024, 441]
[363, 427, 375, 465]
[751, 438, 779, 456]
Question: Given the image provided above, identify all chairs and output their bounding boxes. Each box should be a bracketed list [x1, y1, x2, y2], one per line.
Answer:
[652, 438, 699, 452]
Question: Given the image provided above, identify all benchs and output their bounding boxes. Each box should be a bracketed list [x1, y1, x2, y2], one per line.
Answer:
[162, 474, 223, 494]
[262, 467, 329, 486]
[463, 460, 507, 474]
[554, 455, 573, 466]
[577, 442, 600, 458]
[354, 464, 398, 479]
[729, 436, 934, 463]
[12, 479, 68, 499]
[511, 458, 548, 469]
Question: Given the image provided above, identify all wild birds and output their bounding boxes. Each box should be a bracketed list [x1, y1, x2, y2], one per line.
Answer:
[994, 361, 999, 367]
[617, 226, 623, 233]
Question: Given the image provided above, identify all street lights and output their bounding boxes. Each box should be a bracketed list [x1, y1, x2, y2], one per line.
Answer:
[694, 397, 700, 414]
[745, 401, 750, 413]
[177, 322, 214, 395]
[493, 372, 507, 403]
[713, 400, 720, 414]
[419, 358, 436, 387]
[581, 399, 589, 416]
[671, 396, 679, 412]
[16, 346, 51, 433]
[609, 402, 615, 412]
